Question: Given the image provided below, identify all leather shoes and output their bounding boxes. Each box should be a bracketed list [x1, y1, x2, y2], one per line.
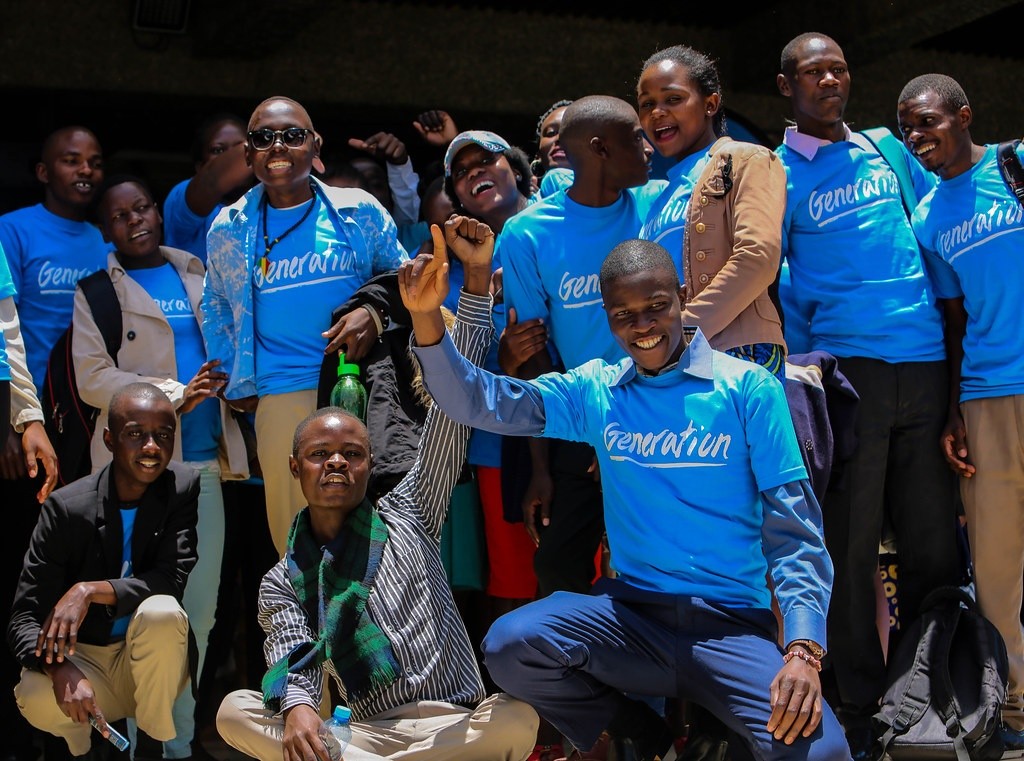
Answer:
[615, 698, 674, 761]
[676, 730, 715, 761]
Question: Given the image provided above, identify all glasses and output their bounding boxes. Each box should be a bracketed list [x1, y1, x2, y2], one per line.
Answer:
[247, 127, 315, 151]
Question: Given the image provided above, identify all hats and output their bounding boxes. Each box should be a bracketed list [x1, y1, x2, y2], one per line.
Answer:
[444, 130, 511, 178]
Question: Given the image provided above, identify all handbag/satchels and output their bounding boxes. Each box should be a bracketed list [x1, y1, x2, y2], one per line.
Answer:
[40, 269, 123, 487]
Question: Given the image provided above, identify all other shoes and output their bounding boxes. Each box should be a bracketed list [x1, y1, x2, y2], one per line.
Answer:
[133, 727, 164, 761]
[44, 735, 68, 761]
[1001, 721, 1024, 749]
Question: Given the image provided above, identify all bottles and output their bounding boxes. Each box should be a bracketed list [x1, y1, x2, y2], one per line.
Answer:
[330, 353, 367, 424]
[310, 705, 352, 761]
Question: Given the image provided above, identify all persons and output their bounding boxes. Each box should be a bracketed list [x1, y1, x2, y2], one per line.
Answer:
[72, 182, 260, 761]
[896, 74, 1024, 752]
[310, 44, 889, 760]
[6, 382, 201, 761]
[201, 97, 410, 722]
[397, 224, 854, 761]
[774, 32, 972, 761]
[215, 214, 540, 761]
[162, 112, 279, 739]
[0, 126, 117, 761]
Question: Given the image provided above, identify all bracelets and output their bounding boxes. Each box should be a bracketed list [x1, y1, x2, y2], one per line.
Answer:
[783, 651, 822, 672]
[788, 640, 823, 659]
[362, 303, 383, 336]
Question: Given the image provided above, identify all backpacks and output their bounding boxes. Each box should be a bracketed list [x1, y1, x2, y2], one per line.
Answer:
[867, 583, 1007, 761]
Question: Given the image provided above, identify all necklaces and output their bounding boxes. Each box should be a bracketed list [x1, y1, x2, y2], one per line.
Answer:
[257, 187, 316, 277]
